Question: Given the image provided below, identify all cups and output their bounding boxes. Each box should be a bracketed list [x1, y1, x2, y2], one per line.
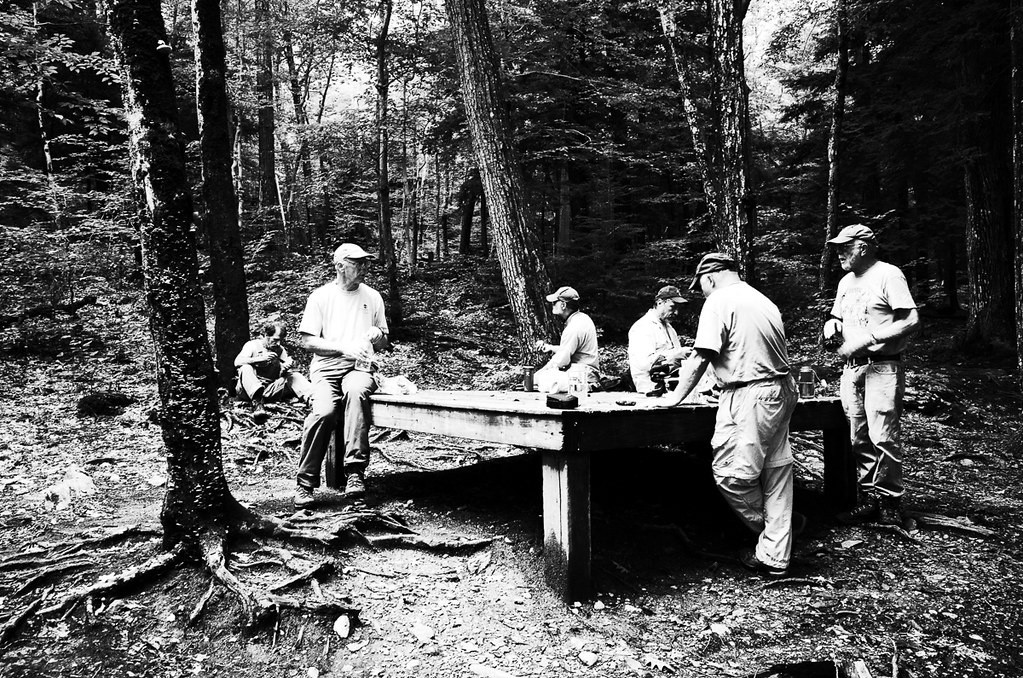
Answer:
[568, 368, 587, 404]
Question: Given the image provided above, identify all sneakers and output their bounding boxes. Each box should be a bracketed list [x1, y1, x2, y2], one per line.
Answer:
[877, 496, 903, 526]
[294, 487, 314, 508]
[836, 499, 876, 524]
[345, 474, 366, 499]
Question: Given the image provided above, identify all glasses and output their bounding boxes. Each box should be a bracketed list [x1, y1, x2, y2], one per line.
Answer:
[834, 243, 858, 250]
[346, 259, 371, 269]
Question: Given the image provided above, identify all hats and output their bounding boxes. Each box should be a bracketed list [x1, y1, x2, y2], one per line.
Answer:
[655, 285, 689, 303]
[689, 253, 736, 292]
[546, 286, 579, 302]
[335, 243, 375, 259]
[826, 224, 876, 249]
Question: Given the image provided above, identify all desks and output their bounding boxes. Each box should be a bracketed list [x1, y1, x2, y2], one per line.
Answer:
[325, 389, 858, 607]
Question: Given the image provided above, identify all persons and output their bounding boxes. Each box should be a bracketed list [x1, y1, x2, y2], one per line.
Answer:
[823, 224, 920, 524]
[292, 243, 389, 508]
[650, 254, 796, 579]
[628, 286, 719, 403]
[234, 323, 315, 422]
[523, 286, 600, 394]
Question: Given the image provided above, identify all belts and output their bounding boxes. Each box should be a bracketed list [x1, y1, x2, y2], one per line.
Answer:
[848, 354, 900, 367]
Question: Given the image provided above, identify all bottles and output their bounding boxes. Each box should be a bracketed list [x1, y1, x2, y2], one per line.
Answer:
[355, 336, 374, 371]
[798, 365, 816, 399]
[522, 365, 535, 391]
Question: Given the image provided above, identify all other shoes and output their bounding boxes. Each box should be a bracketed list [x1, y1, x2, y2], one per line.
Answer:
[735, 559, 786, 580]
[254, 404, 266, 417]
